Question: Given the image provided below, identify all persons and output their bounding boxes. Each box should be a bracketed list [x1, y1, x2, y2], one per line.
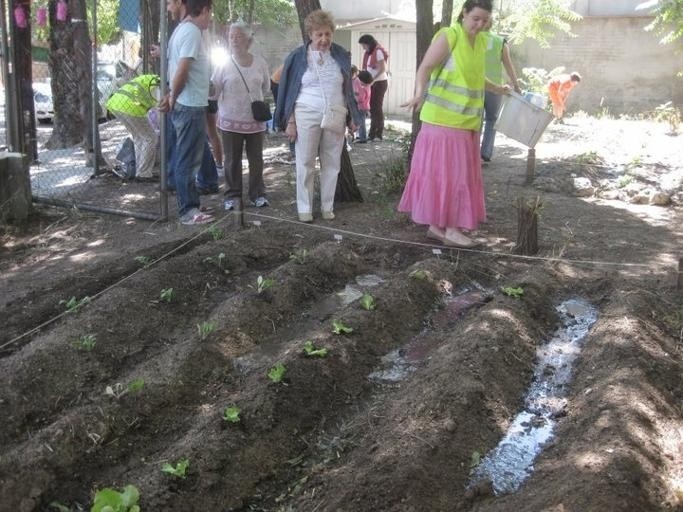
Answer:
[269, 65, 283, 106]
[548, 72, 580, 125]
[479, 18, 520, 162]
[164, 1, 218, 195]
[105, 73, 160, 183]
[350, 65, 357, 92]
[156, 1, 215, 226]
[272, 10, 361, 223]
[358, 35, 388, 142]
[206, 96, 222, 169]
[399, 0, 509, 249]
[353, 71, 373, 143]
[210, 22, 271, 210]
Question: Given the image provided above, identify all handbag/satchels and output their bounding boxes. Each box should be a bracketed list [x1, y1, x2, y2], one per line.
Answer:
[320, 103, 348, 135]
[251, 100, 272, 122]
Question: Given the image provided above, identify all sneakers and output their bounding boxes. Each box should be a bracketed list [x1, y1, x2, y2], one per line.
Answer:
[198, 206, 216, 214]
[224, 198, 238, 211]
[443, 238, 482, 249]
[251, 197, 269, 207]
[321, 212, 335, 220]
[297, 213, 314, 222]
[426, 229, 445, 243]
[180, 211, 216, 226]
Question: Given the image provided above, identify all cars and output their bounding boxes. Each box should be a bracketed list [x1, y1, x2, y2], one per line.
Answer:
[34, 60, 137, 125]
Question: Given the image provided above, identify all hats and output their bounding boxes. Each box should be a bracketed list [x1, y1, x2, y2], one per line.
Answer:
[359, 70, 373, 84]
